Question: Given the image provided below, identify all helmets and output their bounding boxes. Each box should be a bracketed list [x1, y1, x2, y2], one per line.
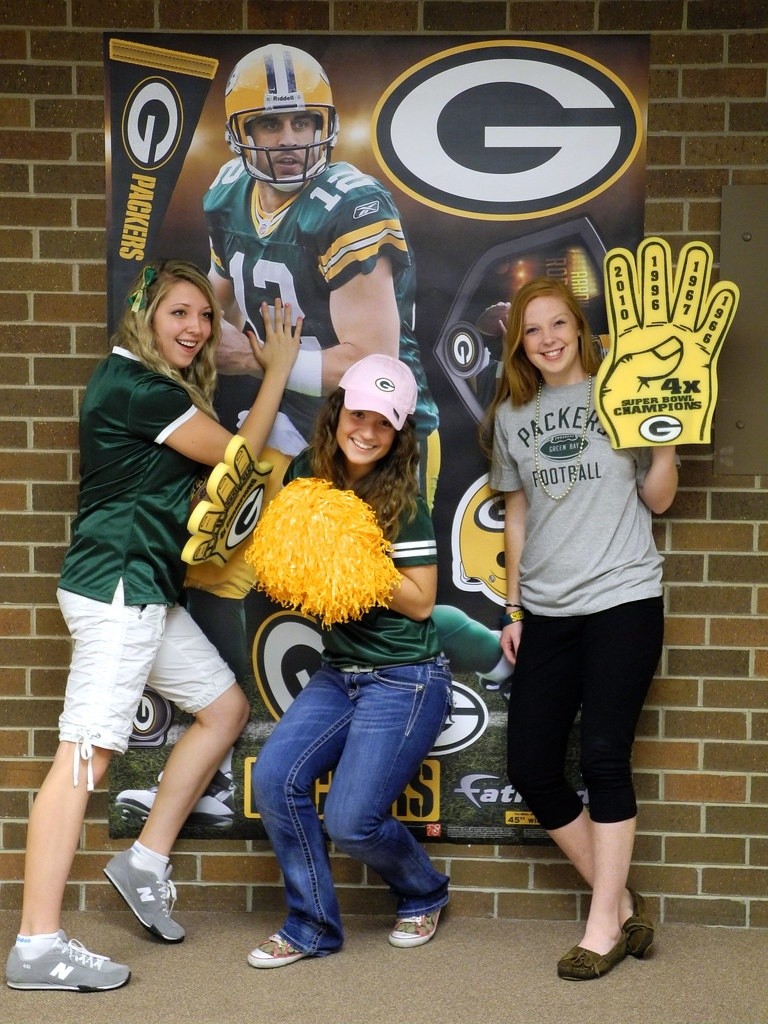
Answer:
[224, 44, 339, 158]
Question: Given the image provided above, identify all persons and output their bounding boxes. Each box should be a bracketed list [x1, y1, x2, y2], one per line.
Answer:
[477, 277, 679, 981]
[248, 355, 450, 969]
[115, 45, 518, 832]
[6, 261, 304, 992]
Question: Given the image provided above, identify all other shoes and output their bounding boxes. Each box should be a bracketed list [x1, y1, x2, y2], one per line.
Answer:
[621, 885, 655, 960]
[557, 929, 628, 981]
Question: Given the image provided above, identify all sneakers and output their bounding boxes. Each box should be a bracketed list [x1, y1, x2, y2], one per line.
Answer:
[103, 848, 186, 944]
[388, 908, 441, 948]
[113, 762, 235, 830]
[5, 929, 132, 993]
[478, 630, 515, 690]
[248, 933, 308, 969]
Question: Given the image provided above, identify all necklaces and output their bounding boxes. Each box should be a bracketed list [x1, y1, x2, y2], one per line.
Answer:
[535, 374, 592, 500]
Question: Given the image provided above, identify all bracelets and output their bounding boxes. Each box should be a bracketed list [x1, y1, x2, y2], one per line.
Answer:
[501, 609, 525, 626]
[504, 604, 523, 607]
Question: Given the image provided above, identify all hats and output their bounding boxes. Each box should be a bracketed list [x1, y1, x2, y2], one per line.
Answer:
[338, 353, 418, 431]
